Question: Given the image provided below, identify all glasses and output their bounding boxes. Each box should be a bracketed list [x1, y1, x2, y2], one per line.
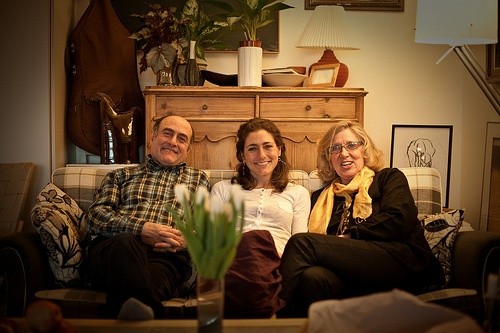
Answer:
[327, 142, 363, 154]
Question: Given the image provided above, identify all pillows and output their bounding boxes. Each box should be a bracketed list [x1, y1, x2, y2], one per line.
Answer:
[414, 209, 468, 284]
[30, 182, 89, 288]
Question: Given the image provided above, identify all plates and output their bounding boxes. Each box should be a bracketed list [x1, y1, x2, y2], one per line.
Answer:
[262, 72, 307, 87]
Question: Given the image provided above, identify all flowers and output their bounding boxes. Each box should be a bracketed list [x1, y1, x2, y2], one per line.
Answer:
[170, 184, 244, 275]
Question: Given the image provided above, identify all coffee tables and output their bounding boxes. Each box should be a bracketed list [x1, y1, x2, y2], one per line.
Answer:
[0, 316, 309, 333]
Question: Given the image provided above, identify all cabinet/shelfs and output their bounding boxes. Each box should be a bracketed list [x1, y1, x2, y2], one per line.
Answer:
[144, 82, 371, 171]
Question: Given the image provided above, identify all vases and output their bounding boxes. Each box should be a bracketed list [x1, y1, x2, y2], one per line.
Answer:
[196, 270, 227, 332]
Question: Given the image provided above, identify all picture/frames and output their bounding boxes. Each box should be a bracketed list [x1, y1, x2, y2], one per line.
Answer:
[110, 0, 281, 55]
[307, 64, 340, 89]
[484, 0, 500, 84]
[477, 121, 500, 235]
[389, 123, 454, 209]
[304, 0, 405, 12]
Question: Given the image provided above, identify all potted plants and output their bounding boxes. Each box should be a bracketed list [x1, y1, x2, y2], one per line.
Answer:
[131, 0, 296, 85]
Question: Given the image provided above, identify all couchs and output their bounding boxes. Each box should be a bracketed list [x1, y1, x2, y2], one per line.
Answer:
[0, 165, 500, 333]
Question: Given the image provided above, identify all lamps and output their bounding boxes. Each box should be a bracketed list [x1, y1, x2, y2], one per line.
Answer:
[295, 6, 361, 87]
[413, 0, 499, 65]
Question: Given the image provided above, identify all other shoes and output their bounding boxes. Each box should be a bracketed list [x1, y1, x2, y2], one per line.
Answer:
[119, 298, 153, 321]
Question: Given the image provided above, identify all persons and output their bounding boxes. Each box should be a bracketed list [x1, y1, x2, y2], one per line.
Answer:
[84, 116, 211, 320]
[209, 118, 310, 320]
[278, 121, 446, 318]
